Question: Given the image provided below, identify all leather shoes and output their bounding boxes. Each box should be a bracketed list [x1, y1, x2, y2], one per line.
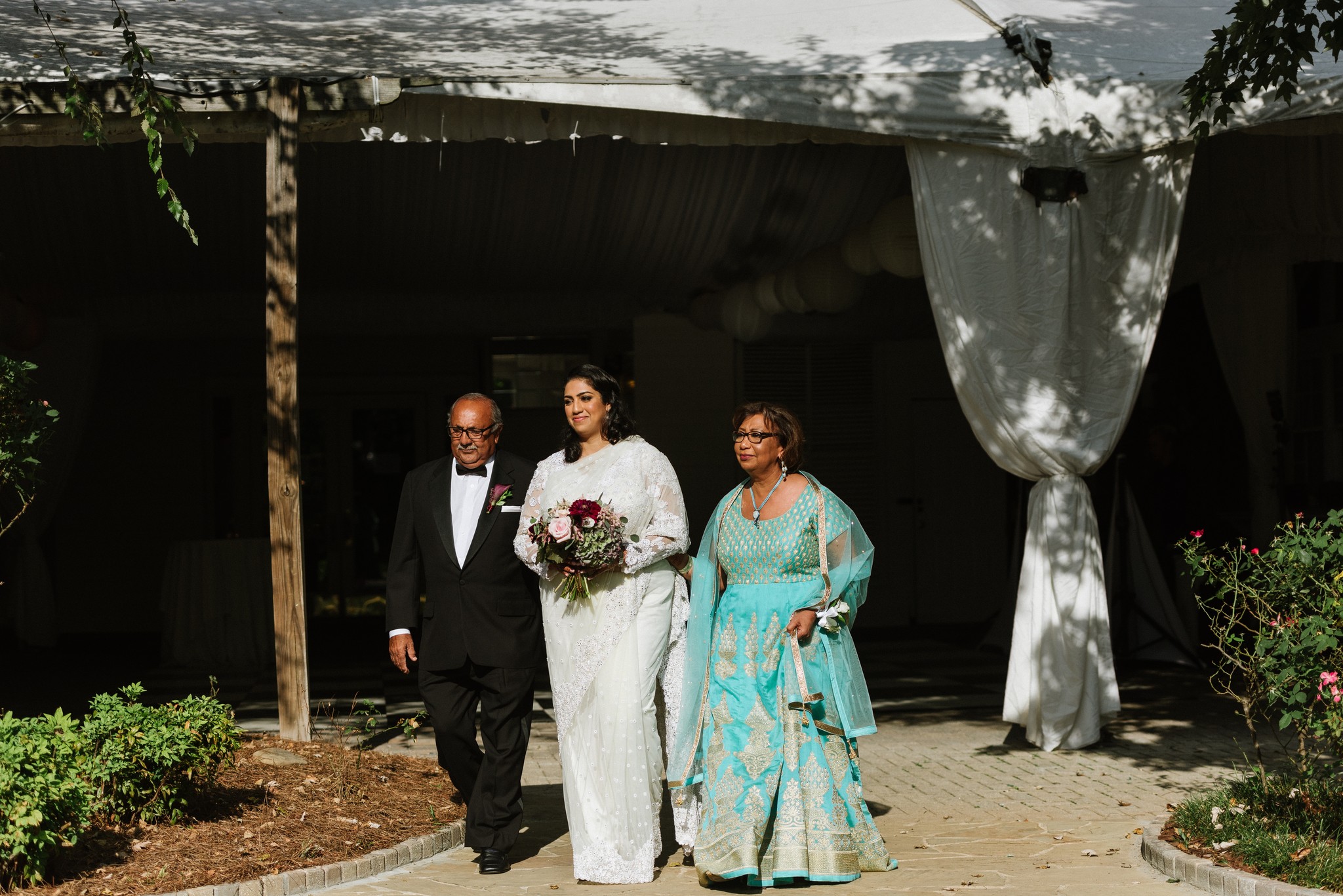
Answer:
[480, 845, 510, 874]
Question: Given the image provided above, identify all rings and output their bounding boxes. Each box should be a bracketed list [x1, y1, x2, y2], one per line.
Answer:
[802, 640, 805, 642]
[800, 638, 803, 642]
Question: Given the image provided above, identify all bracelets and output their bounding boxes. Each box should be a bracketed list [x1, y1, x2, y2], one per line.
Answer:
[678, 554, 693, 574]
[814, 599, 850, 634]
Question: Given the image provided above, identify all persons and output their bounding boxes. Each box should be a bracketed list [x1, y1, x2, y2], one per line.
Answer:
[0, 248, 64, 350]
[1141, 422, 1178, 467]
[513, 364, 708, 884]
[385, 393, 544, 875]
[666, 400, 898, 887]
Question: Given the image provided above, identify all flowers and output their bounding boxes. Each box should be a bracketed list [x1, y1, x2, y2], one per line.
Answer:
[518, 491, 640, 602]
[486, 484, 515, 513]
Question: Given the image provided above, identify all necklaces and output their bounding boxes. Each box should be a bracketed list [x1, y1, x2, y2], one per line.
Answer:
[748, 472, 784, 528]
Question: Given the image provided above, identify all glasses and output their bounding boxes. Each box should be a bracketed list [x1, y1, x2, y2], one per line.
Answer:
[445, 421, 499, 439]
[733, 430, 778, 444]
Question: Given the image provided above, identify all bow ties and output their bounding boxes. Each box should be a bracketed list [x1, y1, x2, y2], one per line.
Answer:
[455, 463, 488, 477]
